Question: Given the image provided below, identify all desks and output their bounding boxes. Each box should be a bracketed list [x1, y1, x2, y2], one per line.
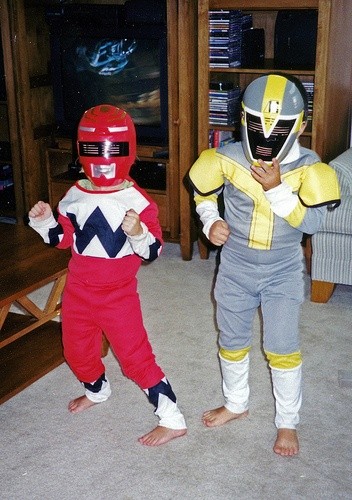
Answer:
[0, 222, 109, 407]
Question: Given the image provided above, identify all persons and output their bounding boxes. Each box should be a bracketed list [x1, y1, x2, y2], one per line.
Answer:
[182, 76, 341, 456]
[29, 103, 188, 445]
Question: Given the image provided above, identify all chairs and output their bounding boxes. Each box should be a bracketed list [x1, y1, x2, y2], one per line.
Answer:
[309, 143, 352, 304]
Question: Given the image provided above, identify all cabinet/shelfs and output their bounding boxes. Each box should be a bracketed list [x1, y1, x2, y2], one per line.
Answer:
[9, 0, 180, 245]
[195, 0, 352, 260]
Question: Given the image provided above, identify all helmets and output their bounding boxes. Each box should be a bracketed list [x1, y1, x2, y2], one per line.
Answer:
[241, 75, 306, 169]
[77, 105, 137, 188]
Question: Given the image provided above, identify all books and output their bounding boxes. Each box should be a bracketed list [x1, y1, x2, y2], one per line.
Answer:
[208, 8, 253, 67]
[298, 81, 314, 132]
[207, 129, 236, 149]
[208, 80, 241, 125]
